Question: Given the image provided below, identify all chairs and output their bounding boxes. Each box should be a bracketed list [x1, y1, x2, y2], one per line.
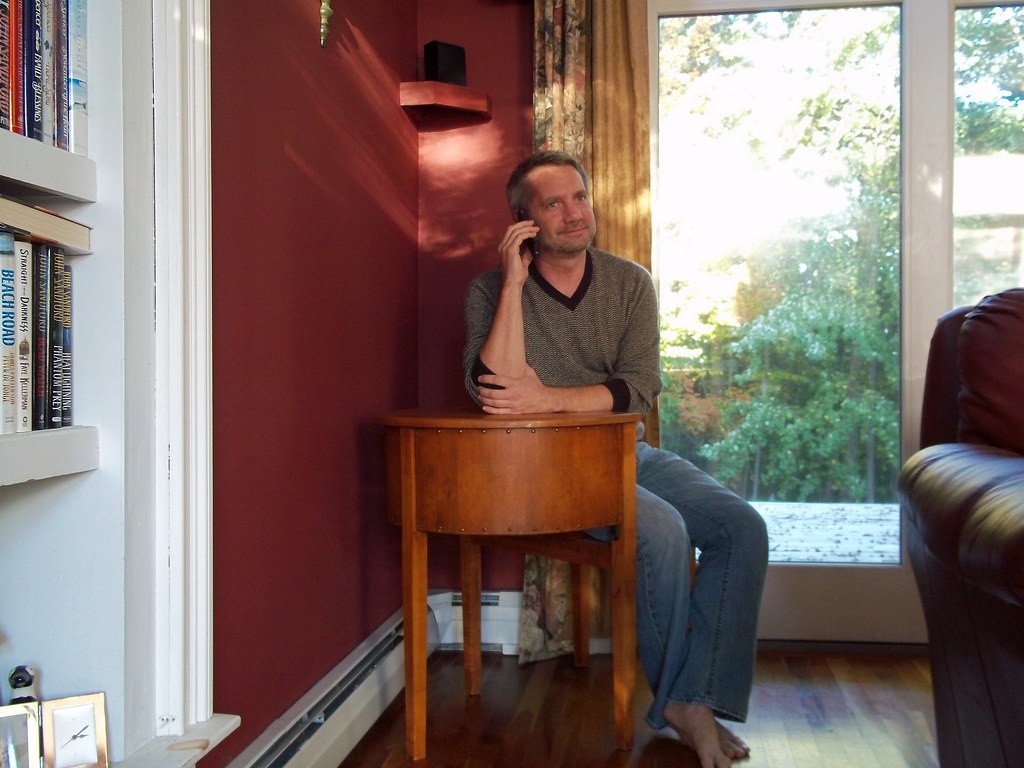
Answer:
[457, 530, 699, 697]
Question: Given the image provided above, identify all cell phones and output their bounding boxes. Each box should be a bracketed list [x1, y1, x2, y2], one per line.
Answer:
[517, 209, 539, 259]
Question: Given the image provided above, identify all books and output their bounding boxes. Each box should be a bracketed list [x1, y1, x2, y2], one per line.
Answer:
[0, 0, 88, 157]
[0, 194, 94, 435]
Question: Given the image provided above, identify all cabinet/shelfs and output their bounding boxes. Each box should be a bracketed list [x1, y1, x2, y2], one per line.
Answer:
[0, 127, 101, 485]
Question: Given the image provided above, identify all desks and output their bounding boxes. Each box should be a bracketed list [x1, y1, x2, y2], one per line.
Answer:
[378, 407, 642, 762]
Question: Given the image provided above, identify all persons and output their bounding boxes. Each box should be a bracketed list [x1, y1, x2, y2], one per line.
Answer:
[464, 150, 769, 768]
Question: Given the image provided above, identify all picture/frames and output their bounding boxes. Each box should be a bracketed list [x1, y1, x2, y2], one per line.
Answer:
[0, 701, 40, 768]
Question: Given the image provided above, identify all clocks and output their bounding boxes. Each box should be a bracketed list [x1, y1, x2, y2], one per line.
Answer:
[41, 691, 109, 768]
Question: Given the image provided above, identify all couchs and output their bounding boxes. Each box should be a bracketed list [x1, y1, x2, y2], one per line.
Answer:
[894, 289, 1024, 768]
